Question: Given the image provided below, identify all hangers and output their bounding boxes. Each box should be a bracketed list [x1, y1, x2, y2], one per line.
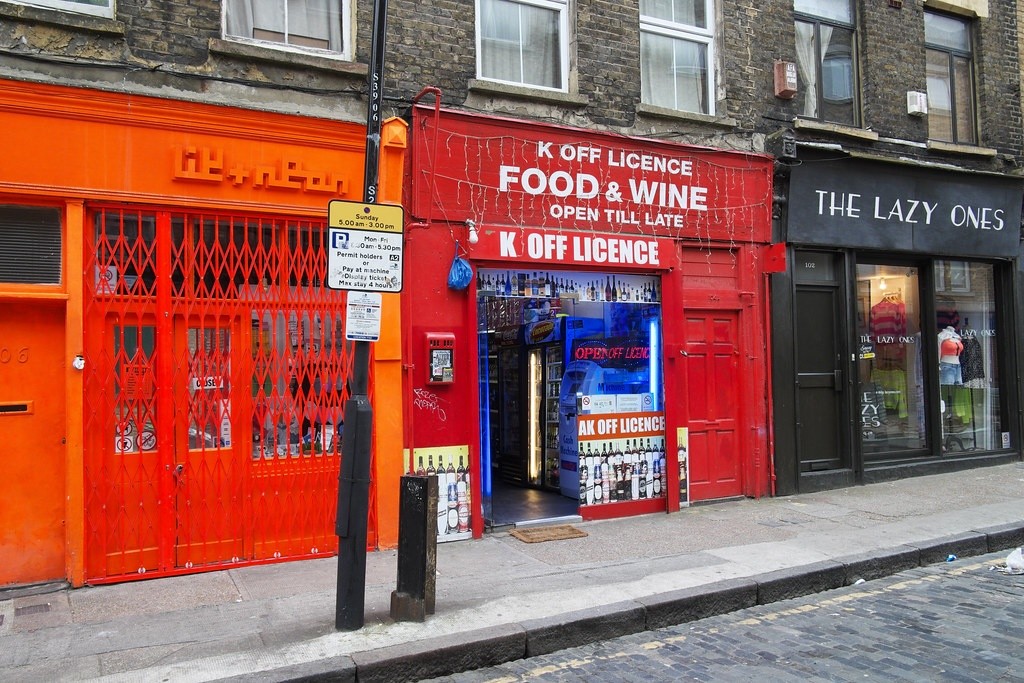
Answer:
[876, 360, 903, 371]
[880, 293, 902, 305]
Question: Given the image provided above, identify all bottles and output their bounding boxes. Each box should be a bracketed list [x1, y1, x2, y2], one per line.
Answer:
[405, 454, 472, 536]
[676, 437, 688, 502]
[578, 438, 667, 506]
[475, 270, 661, 303]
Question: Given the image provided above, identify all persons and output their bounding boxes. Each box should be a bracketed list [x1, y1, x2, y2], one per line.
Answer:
[937, 326, 964, 385]
[960, 328, 985, 386]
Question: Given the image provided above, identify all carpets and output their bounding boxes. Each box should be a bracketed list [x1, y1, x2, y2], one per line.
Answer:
[508, 524, 589, 544]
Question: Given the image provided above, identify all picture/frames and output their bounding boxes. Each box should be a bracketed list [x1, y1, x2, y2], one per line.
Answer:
[857, 298, 866, 328]
